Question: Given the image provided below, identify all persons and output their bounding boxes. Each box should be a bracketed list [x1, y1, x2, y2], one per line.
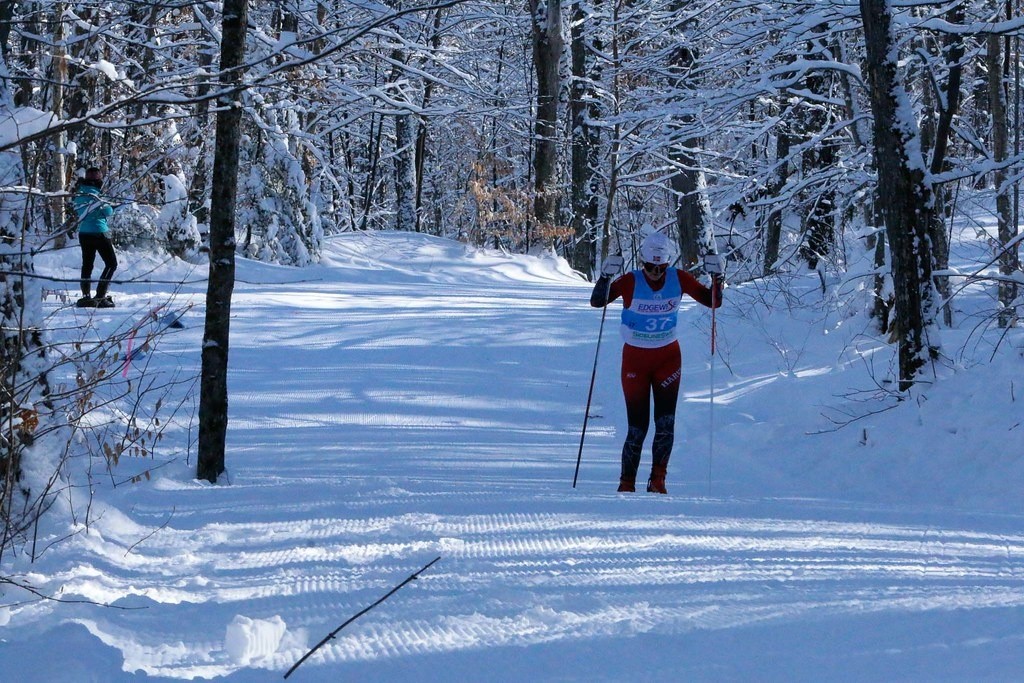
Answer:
[590, 234, 725, 495]
[71, 167, 117, 308]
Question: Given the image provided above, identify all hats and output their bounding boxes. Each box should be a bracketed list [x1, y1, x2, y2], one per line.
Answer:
[86, 168, 103, 180]
[640, 233, 671, 265]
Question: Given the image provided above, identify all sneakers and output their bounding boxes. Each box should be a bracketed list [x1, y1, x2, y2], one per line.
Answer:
[647, 463, 667, 494]
[617, 478, 635, 492]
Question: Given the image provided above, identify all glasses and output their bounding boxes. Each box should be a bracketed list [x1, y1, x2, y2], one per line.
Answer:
[641, 260, 668, 273]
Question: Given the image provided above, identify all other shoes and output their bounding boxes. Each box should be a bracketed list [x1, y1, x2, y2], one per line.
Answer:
[77, 298, 97, 307]
[93, 296, 115, 307]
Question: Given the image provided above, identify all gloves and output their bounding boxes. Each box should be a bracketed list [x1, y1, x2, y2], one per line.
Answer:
[600, 250, 623, 278]
[703, 253, 726, 286]
